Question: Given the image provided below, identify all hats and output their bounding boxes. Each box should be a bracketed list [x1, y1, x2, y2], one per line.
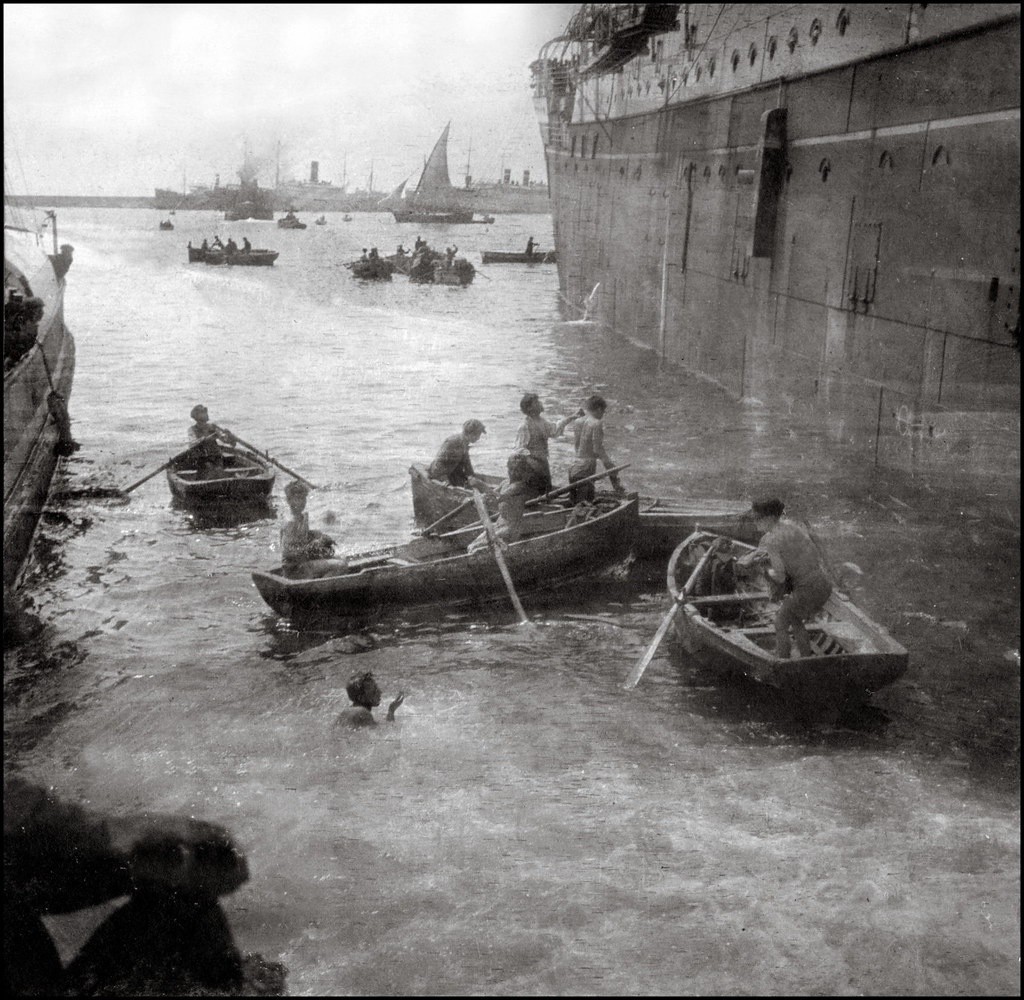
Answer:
[751, 497, 785, 516]
[191, 404, 207, 418]
[463, 419, 487, 435]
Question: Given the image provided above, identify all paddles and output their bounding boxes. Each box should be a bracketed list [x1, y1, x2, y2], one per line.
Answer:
[624, 545, 714, 690]
[423, 488, 494, 533]
[468, 474, 529, 623]
[225, 428, 317, 491]
[462, 462, 631, 529]
[123, 432, 219, 495]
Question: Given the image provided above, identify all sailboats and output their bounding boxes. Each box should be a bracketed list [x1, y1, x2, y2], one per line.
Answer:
[378, 119, 495, 224]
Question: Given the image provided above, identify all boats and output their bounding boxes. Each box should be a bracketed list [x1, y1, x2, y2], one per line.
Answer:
[481, 251, 556, 264]
[251, 491, 640, 623]
[666, 522, 909, 708]
[409, 461, 787, 554]
[349, 251, 475, 286]
[155, 133, 552, 230]
[167, 442, 276, 505]
[4, 128, 77, 595]
[528, 4, 1020, 349]
[188, 241, 279, 266]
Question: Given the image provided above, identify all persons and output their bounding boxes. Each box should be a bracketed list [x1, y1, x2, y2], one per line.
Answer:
[567, 396, 614, 505]
[466, 448, 532, 553]
[187, 406, 236, 478]
[202, 235, 251, 253]
[514, 394, 580, 494]
[340, 671, 404, 728]
[361, 247, 378, 261]
[52, 245, 74, 279]
[397, 235, 458, 266]
[429, 418, 486, 490]
[751, 496, 833, 659]
[5, 287, 45, 363]
[279, 479, 348, 579]
[526, 237, 538, 254]
[693, 538, 738, 621]
